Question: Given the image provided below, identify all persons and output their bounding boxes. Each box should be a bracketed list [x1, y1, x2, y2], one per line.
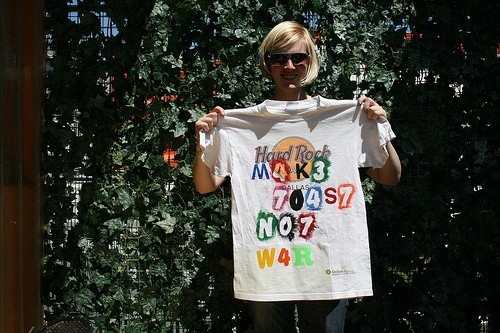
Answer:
[192, 21, 401, 333]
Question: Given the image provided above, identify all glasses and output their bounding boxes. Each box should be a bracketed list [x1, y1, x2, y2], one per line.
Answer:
[269, 51, 309, 65]
